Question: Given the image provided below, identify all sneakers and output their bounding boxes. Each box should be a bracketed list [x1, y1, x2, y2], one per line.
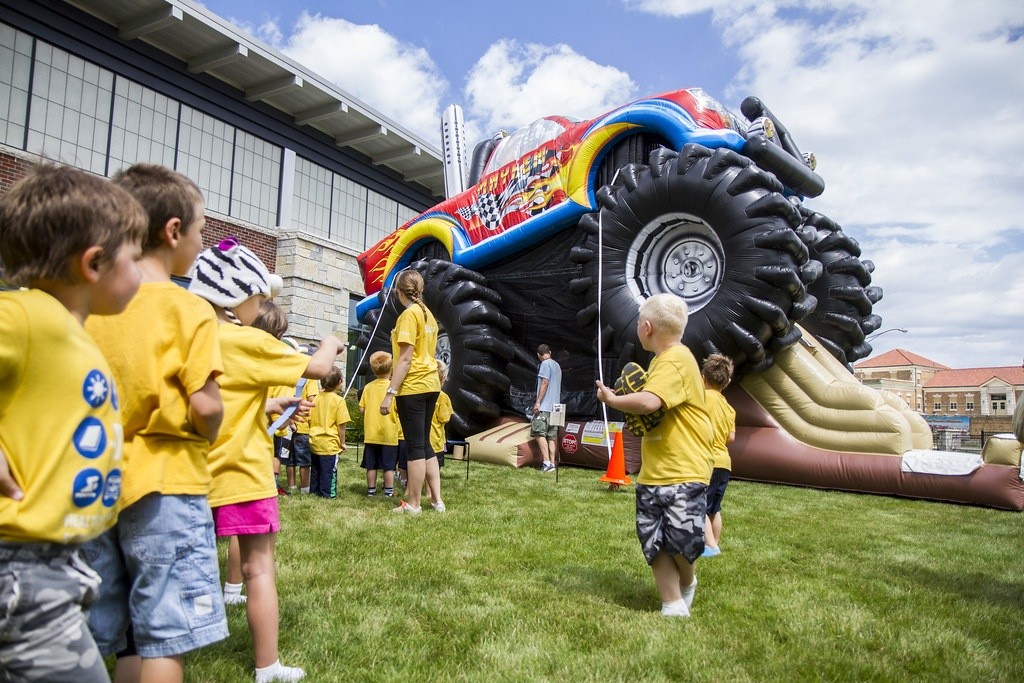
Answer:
[388, 500, 422, 515]
[621, 362, 666, 422]
[614, 377, 647, 437]
[431, 501, 446, 511]
[542, 462, 555, 472]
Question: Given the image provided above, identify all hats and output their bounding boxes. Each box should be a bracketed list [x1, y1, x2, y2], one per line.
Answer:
[188, 237, 283, 325]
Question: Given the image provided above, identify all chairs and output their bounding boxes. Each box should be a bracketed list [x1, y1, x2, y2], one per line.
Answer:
[447, 440, 470, 481]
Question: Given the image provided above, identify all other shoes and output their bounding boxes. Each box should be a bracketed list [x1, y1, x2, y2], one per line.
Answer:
[367, 490, 376, 496]
[278, 487, 288, 497]
[393, 470, 401, 480]
[401, 479, 407, 487]
[301, 490, 310, 495]
[385, 493, 393, 497]
[287, 486, 301, 493]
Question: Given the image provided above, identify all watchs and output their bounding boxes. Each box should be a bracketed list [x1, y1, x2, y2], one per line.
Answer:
[386, 387, 398, 395]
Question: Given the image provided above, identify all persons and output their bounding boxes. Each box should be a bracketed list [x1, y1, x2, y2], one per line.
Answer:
[700, 351, 736, 559]
[1, 159, 228, 683]
[531, 343, 561, 473]
[596, 292, 713, 618]
[359, 271, 455, 516]
[191, 241, 352, 683]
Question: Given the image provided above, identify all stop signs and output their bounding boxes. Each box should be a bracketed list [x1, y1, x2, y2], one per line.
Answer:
[562, 434, 577, 453]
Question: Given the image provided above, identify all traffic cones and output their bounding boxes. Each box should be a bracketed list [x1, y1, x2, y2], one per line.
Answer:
[600, 431, 631, 486]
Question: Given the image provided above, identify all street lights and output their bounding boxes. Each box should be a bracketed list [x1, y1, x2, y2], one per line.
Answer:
[851, 327, 908, 378]
